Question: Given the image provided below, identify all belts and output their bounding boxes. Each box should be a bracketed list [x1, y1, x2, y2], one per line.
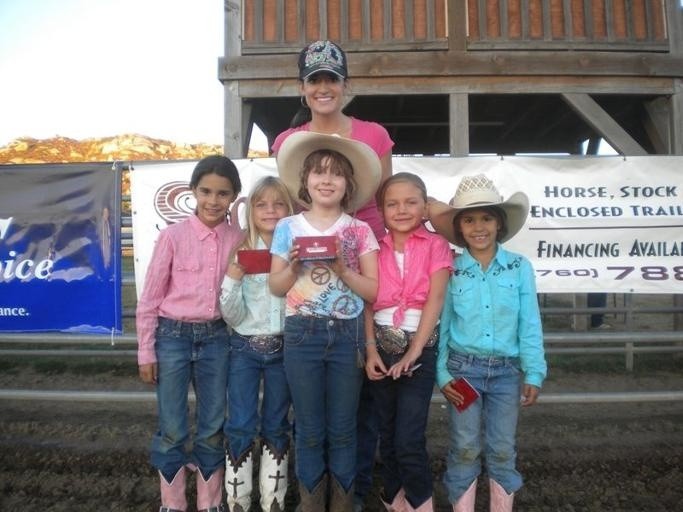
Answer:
[377, 326, 438, 354]
[236, 335, 283, 355]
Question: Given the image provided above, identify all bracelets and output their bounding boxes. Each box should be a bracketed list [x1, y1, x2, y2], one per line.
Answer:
[364, 340, 377, 347]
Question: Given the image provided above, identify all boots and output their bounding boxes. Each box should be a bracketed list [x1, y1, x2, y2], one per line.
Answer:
[158, 444, 290, 511]
[296, 474, 516, 512]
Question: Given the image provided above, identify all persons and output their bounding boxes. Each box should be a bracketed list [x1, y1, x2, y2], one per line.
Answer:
[270, 40, 394, 512]
[364, 172, 454, 512]
[270, 132, 384, 512]
[426, 176, 547, 512]
[220, 176, 295, 512]
[136, 156, 247, 512]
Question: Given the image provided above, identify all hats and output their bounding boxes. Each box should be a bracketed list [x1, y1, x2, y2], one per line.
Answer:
[277, 131, 383, 215]
[299, 40, 347, 81]
[430, 175, 530, 246]
